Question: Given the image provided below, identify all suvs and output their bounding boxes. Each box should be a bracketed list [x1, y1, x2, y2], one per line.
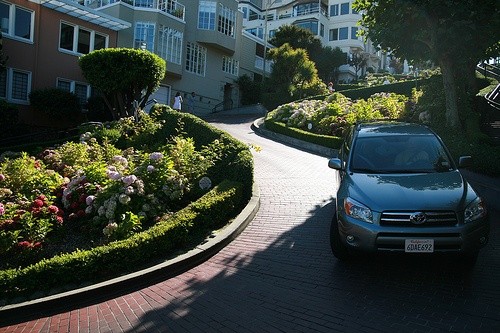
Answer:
[327, 120, 488, 264]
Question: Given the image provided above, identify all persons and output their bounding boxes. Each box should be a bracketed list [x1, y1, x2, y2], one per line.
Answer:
[188, 92, 195, 114]
[182, 93, 187, 113]
[173, 92, 183, 112]
[327, 82, 334, 93]
